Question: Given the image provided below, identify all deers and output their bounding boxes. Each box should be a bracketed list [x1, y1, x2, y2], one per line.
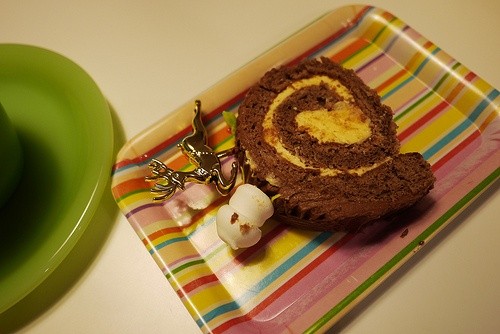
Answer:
[145, 99, 239, 204]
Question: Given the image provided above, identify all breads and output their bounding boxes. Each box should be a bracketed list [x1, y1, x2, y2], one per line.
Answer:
[235, 56, 436, 232]
